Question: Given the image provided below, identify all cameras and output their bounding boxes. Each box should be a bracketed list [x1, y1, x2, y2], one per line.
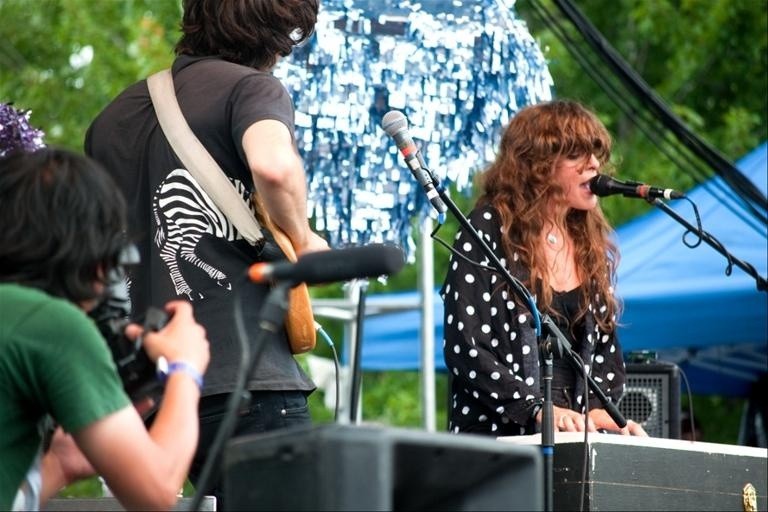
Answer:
[107, 307, 171, 403]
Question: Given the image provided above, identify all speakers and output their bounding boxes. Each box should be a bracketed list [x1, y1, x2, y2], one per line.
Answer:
[608, 363, 685, 439]
[224, 417, 543, 512]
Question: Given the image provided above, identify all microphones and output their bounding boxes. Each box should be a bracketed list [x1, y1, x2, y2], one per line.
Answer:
[382, 109, 448, 214]
[590, 174, 682, 199]
[246, 245, 404, 287]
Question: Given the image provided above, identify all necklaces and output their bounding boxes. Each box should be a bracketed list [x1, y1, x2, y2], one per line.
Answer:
[543, 220, 562, 244]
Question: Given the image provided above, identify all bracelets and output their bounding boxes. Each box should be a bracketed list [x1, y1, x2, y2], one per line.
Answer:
[157, 362, 205, 391]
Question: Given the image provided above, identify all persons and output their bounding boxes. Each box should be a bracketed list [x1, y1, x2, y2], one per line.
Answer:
[84, 1, 338, 511]
[438, 99, 653, 443]
[0, 146, 213, 511]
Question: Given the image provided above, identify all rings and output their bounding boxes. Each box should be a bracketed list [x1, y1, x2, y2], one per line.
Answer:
[557, 412, 567, 427]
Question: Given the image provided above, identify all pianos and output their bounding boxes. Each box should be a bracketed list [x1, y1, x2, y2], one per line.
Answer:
[496, 426, 767, 510]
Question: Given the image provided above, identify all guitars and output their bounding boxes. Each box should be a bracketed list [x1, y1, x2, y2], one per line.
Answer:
[249, 190, 316, 354]
